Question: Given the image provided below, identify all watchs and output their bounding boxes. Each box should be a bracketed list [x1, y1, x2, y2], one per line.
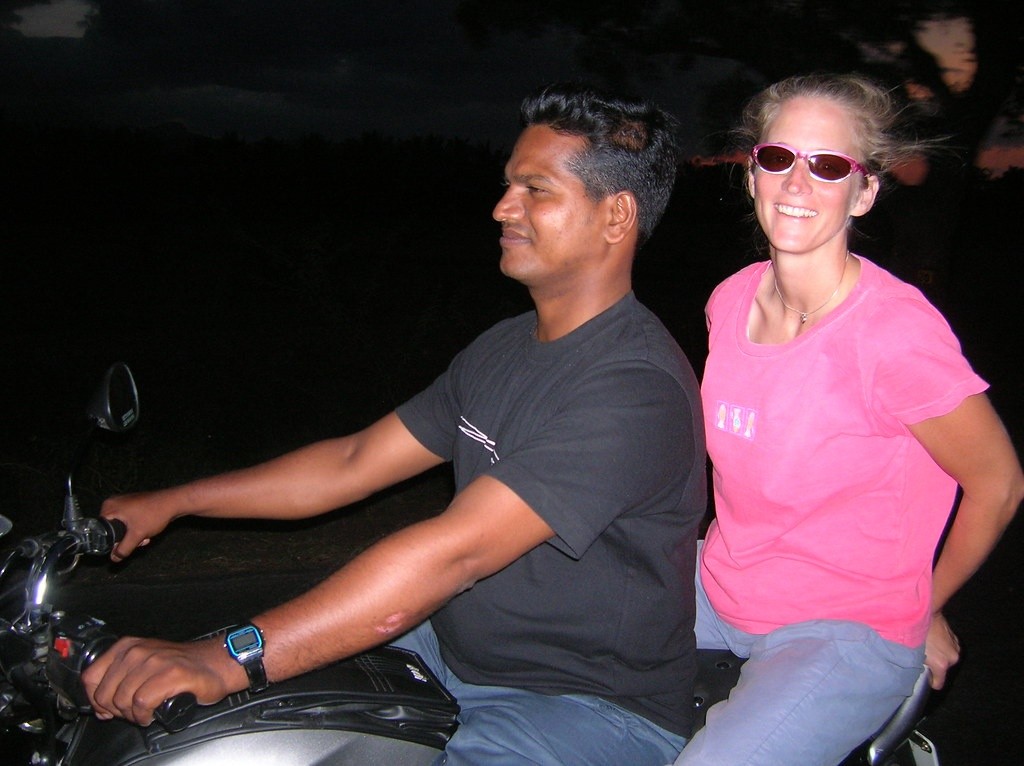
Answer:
[225, 619, 269, 694]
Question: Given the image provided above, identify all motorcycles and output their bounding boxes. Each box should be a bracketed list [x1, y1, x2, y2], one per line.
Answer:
[0, 361, 964, 766]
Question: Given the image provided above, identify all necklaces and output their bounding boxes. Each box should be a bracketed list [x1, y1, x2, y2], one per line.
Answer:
[769, 250, 849, 323]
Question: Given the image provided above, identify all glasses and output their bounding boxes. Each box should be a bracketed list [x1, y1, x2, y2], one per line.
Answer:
[753, 143, 881, 183]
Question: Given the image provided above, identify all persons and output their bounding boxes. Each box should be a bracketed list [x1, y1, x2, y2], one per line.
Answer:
[83, 85, 705, 766]
[674, 78, 1023, 766]
